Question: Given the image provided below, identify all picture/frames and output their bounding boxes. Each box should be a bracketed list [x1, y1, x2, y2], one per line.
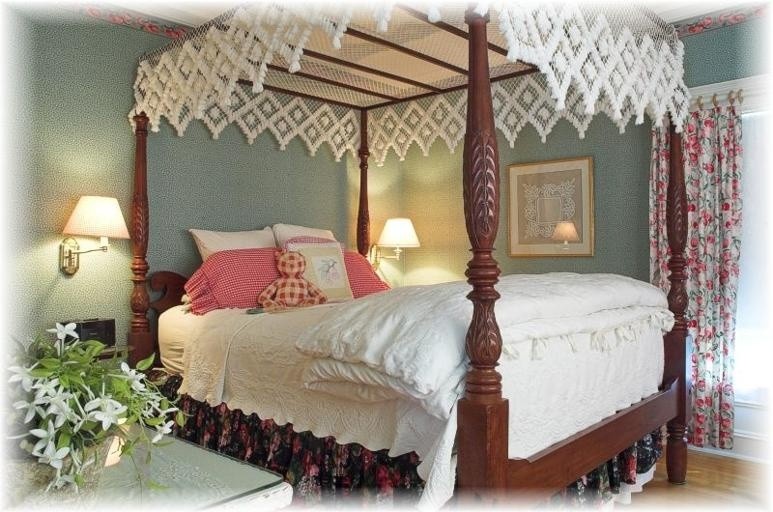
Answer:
[508, 154, 594, 258]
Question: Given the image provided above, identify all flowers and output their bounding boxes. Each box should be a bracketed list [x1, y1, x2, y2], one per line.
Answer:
[5, 321, 194, 498]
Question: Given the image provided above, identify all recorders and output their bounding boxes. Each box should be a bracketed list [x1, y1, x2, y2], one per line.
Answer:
[61, 316, 115, 347]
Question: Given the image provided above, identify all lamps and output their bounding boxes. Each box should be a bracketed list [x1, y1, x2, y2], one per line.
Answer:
[370, 218, 421, 272]
[551, 220, 581, 252]
[59, 195, 131, 276]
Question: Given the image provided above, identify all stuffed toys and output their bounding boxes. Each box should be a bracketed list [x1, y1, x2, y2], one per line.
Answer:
[255, 249, 328, 311]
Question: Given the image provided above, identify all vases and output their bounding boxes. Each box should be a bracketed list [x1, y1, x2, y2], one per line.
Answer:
[4, 432, 116, 504]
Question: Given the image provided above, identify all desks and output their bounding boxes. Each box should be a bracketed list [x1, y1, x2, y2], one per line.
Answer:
[5, 422, 294, 504]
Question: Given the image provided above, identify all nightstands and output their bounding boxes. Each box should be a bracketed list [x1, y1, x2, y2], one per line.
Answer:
[93, 345, 137, 369]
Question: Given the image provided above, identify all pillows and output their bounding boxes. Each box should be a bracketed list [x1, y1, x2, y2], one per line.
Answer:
[286, 236, 354, 303]
[184, 247, 392, 315]
[188, 225, 277, 262]
[272, 222, 336, 247]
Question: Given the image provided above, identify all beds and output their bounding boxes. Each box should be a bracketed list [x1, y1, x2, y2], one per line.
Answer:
[126, 0, 690, 504]
